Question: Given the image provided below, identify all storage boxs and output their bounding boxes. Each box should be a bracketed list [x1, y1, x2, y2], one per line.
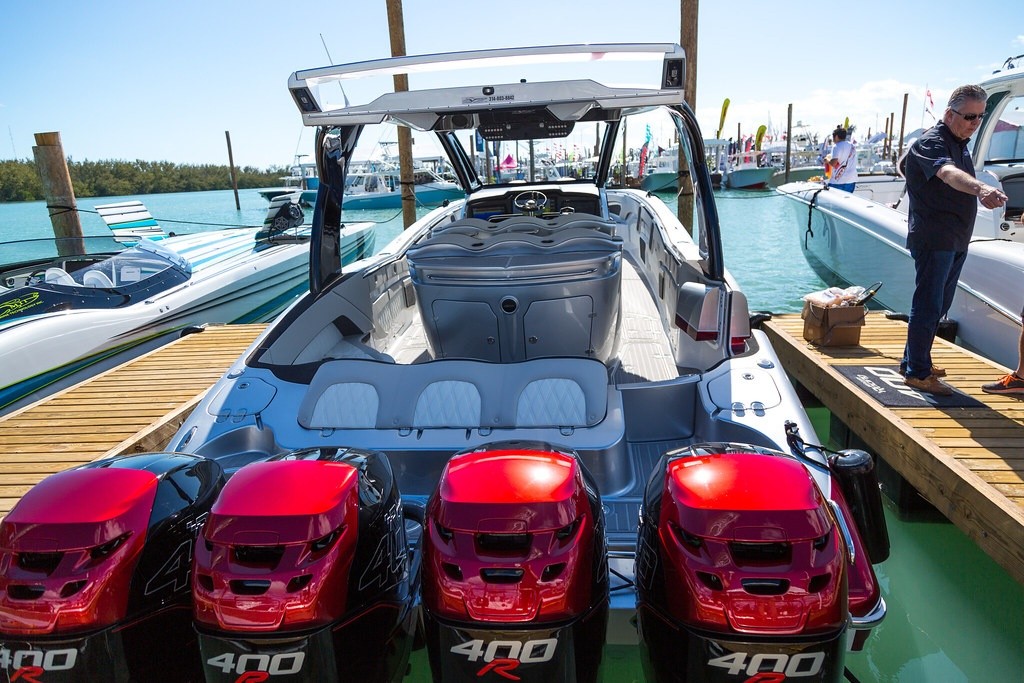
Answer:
[801, 304, 867, 346]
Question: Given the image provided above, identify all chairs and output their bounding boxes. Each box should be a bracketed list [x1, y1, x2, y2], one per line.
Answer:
[45, 268, 74, 285]
[83, 270, 113, 287]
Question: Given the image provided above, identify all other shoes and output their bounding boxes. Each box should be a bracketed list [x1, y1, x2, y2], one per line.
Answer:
[903, 375, 954, 396]
[899, 362, 946, 376]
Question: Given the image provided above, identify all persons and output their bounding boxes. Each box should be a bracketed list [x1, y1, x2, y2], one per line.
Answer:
[823, 128, 858, 193]
[898, 85, 1008, 395]
[892, 152, 897, 164]
[981, 306, 1024, 394]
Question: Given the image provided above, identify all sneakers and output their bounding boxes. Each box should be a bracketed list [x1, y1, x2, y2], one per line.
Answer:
[981, 374, 1024, 394]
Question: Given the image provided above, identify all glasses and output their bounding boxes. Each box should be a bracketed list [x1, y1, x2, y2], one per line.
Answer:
[952, 109, 985, 120]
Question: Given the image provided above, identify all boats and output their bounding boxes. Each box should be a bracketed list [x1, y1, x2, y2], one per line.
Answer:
[0, 191, 378, 419]
[625, 121, 908, 192]
[257, 141, 467, 210]
[775, 55, 1024, 371]
[0, 42, 893, 683]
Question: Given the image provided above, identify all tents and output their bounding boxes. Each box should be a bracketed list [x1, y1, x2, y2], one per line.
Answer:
[495, 155, 517, 171]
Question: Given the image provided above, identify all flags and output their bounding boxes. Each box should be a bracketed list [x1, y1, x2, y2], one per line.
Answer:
[925, 89, 935, 120]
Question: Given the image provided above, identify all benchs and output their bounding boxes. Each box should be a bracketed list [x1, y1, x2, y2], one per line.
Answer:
[406, 212, 623, 362]
